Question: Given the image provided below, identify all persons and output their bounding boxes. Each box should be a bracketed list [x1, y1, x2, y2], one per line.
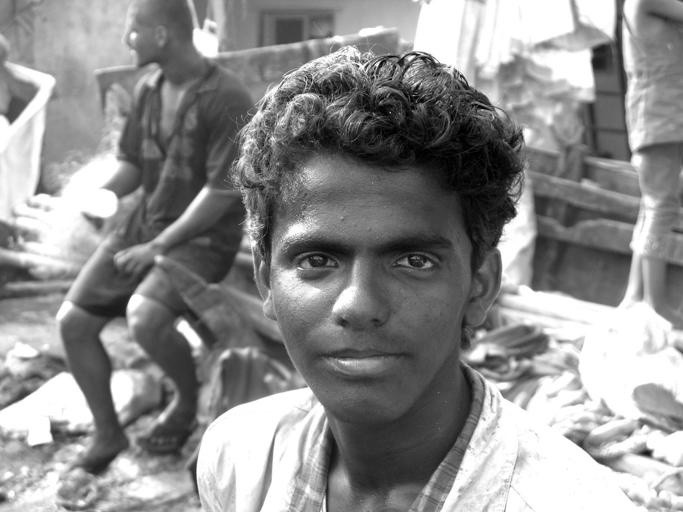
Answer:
[54, 0, 263, 480]
[194, 47, 648, 512]
[0, 35, 13, 131]
[614, 0, 683, 327]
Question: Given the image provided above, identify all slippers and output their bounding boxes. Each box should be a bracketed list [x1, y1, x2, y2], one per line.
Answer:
[59, 452, 113, 483]
[136, 421, 199, 453]
